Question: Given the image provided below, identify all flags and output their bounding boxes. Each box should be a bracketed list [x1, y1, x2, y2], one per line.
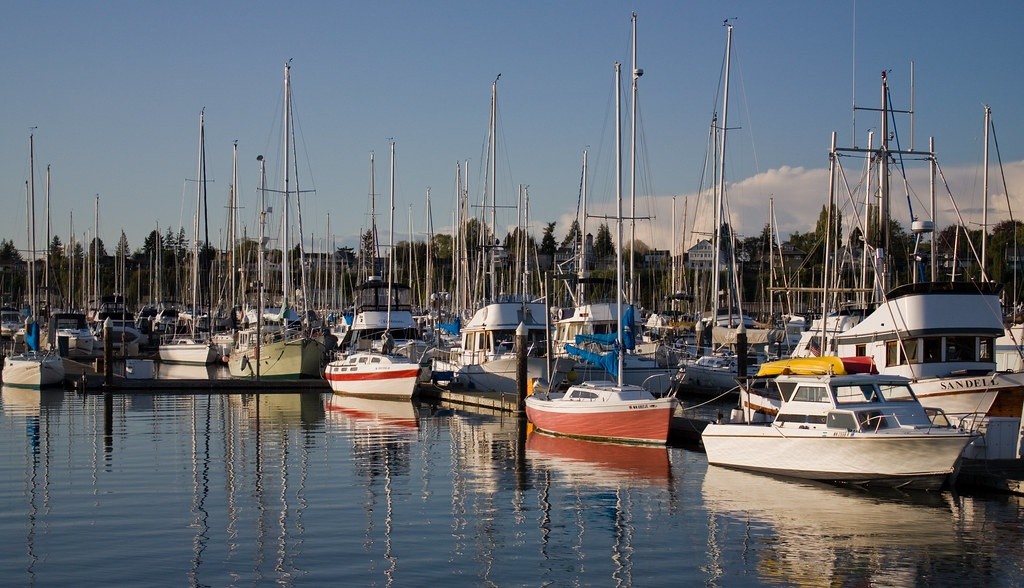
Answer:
[805, 336, 821, 357]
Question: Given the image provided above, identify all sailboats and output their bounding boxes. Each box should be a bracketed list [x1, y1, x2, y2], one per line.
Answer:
[2, 9, 1023, 494]
